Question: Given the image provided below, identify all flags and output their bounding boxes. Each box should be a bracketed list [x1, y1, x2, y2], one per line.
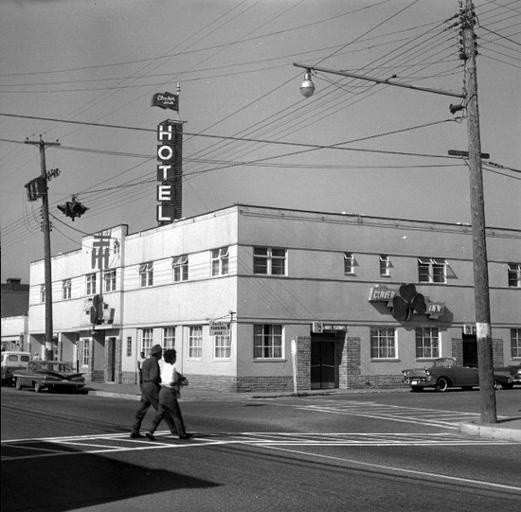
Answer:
[150, 90, 181, 112]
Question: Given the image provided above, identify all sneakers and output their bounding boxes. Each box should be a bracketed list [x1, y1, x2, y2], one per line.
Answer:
[130, 432, 194, 441]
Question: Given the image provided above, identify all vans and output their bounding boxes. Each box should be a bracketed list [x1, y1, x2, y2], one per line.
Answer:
[0, 351, 31, 383]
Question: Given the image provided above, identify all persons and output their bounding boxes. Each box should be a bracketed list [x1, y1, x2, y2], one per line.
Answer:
[129, 344, 192, 441]
[31, 352, 41, 360]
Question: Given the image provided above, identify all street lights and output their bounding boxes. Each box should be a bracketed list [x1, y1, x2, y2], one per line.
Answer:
[292, 61, 497, 423]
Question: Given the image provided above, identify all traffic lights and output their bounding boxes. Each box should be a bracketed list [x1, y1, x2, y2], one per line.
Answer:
[57, 201, 88, 219]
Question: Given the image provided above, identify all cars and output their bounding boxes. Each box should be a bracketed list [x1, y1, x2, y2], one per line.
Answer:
[400, 355, 521, 390]
[14, 359, 85, 391]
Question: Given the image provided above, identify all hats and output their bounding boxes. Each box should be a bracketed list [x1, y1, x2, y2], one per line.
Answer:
[149, 345, 165, 356]
